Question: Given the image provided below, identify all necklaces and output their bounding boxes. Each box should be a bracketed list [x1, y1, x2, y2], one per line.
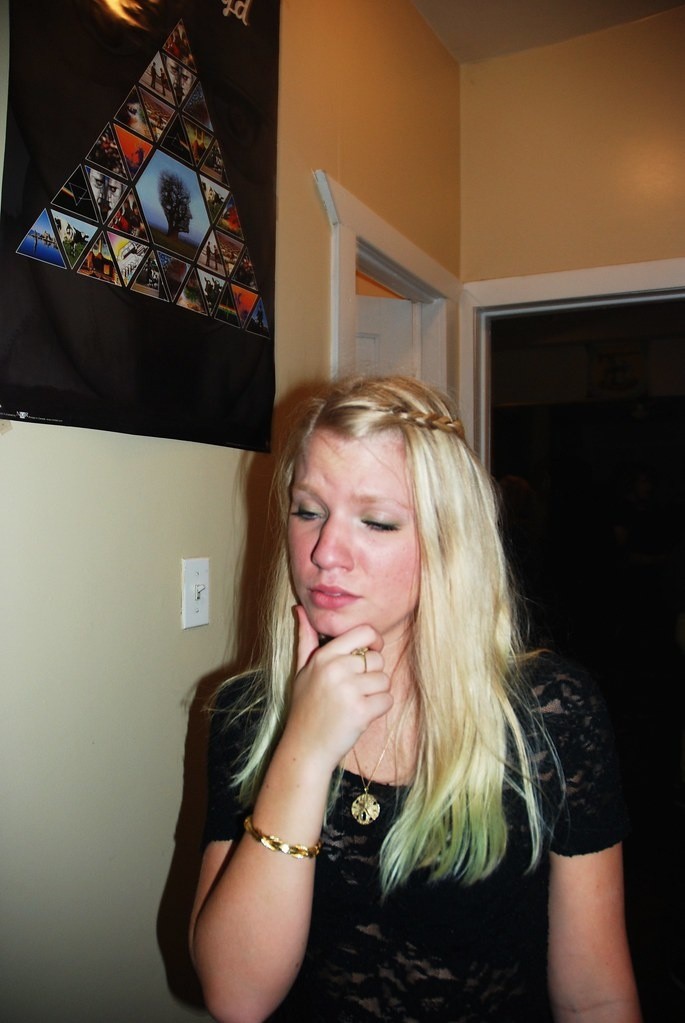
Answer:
[351, 716, 398, 824]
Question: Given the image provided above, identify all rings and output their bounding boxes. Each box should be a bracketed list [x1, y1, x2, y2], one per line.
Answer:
[351, 647, 369, 674]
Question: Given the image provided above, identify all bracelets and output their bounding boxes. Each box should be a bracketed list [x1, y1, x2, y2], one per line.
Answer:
[244, 814, 322, 859]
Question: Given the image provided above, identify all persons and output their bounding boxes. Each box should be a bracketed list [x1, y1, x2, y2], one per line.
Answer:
[156, 378, 642, 1023]
